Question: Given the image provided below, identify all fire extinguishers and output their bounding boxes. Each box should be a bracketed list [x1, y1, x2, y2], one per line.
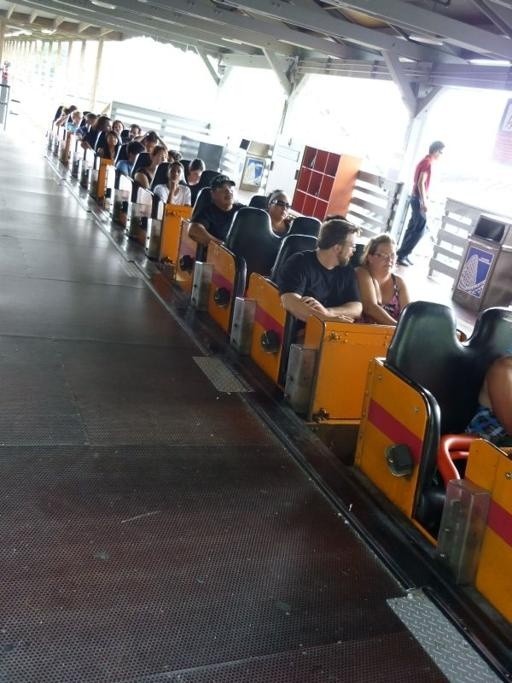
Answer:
[3, 69, 8, 80]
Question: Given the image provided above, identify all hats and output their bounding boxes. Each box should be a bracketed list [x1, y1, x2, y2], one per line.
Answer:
[211, 175, 236, 191]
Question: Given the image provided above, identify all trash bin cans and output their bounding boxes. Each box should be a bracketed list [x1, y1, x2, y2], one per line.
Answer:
[452, 212, 512, 314]
[232, 138, 271, 192]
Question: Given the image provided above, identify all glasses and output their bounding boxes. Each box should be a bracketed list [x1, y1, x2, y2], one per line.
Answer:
[368, 250, 395, 259]
[271, 199, 291, 210]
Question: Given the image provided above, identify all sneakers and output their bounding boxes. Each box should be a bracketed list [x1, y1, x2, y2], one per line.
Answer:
[395, 255, 414, 268]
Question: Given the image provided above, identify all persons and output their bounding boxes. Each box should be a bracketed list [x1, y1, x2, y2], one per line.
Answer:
[54, 105, 141, 159]
[431, 356, 512, 491]
[188, 176, 248, 245]
[353, 235, 410, 325]
[278, 219, 363, 343]
[326, 214, 345, 221]
[395, 140, 444, 267]
[266, 190, 292, 236]
[116, 130, 206, 204]
